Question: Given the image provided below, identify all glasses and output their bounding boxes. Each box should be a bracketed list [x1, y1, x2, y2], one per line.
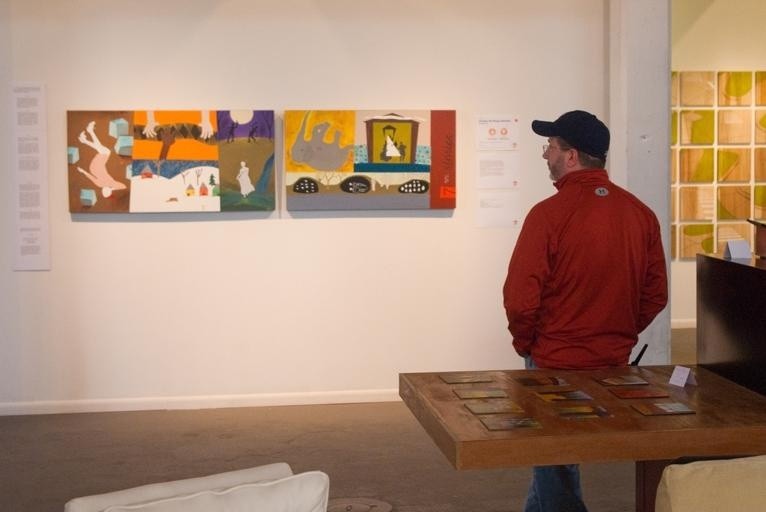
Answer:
[542, 145, 567, 153]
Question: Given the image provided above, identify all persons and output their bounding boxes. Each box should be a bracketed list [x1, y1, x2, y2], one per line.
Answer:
[503, 110, 669, 512]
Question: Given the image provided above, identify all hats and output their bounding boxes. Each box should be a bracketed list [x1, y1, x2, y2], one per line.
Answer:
[532, 111, 609, 158]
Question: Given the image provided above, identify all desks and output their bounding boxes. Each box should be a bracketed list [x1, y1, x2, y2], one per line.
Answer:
[398, 364, 766, 511]
